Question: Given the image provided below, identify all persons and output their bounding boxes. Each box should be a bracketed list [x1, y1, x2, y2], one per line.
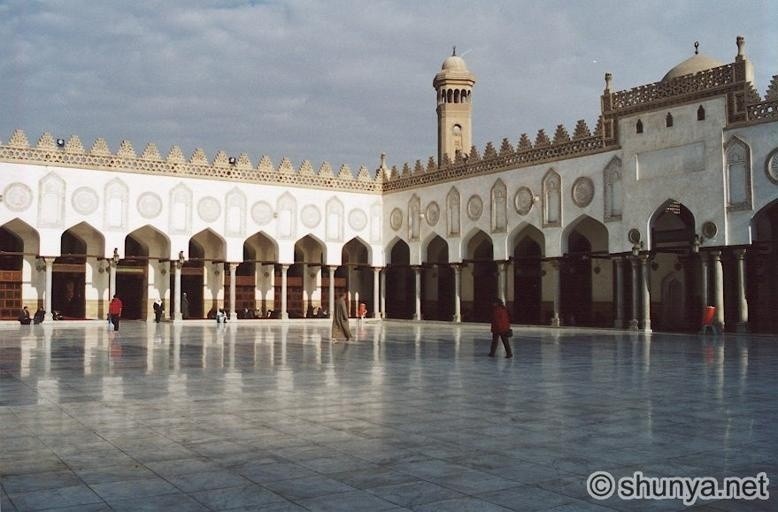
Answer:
[33, 305, 46, 326]
[18, 307, 31, 324]
[332, 293, 354, 343]
[181, 293, 191, 320]
[206, 306, 329, 323]
[153, 297, 164, 323]
[108, 295, 122, 332]
[359, 300, 368, 319]
[487, 296, 513, 358]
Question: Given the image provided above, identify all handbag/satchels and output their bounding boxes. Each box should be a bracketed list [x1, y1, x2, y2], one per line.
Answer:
[506, 329, 512, 337]
